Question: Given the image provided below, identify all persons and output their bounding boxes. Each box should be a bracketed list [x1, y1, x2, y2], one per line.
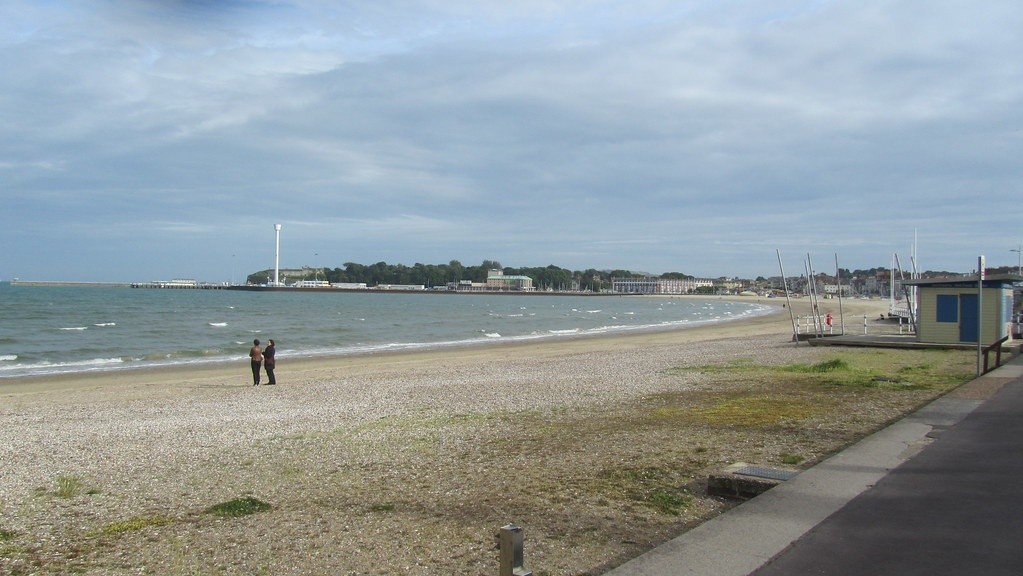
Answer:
[249, 339, 263, 386]
[825, 314, 833, 326]
[262, 339, 276, 385]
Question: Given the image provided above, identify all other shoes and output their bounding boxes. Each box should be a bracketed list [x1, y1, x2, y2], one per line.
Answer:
[268, 382, 275, 385]
[253, 381, 259, 386]
